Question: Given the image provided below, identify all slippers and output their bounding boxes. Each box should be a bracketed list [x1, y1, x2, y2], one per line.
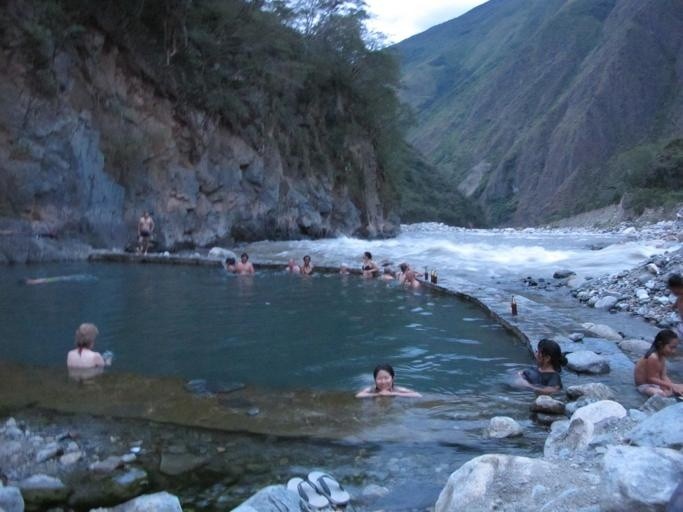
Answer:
[286, 476, 329, 509]
[307, 471, 350, 506]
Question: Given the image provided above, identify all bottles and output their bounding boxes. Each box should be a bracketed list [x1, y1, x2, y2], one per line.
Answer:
[424, 266, 438, 283]
[511, 295, 519, 316]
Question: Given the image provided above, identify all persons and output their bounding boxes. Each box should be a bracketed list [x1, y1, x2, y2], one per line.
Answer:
[135, 211, 155, 255]
[667, 274, 683, 311]
[355, 362, 423, 399]
[501, 339, 568, 394]
[634, 330, 683, 397]
[285, 254, 313, 277]
[17, 274, 95, 286]
[66, 323, 113, 367]
[226, 252, 255, 277]
[339, 252, 424, 290]
